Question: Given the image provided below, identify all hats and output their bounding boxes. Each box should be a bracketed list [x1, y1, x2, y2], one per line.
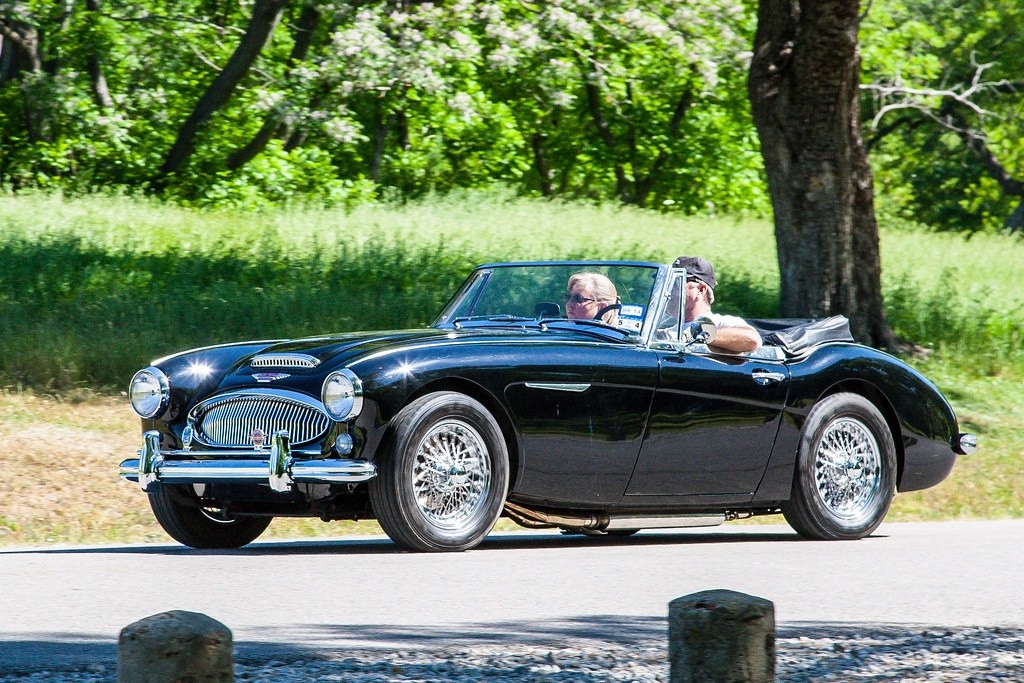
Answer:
[652, 256, 715, 290]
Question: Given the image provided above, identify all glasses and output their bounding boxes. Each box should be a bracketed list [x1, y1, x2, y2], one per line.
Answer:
[563, 291, 600, 303]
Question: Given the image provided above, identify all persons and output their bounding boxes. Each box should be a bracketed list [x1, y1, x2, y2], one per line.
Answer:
[637, 255, 763, 354]
[561, 271, 621, 330]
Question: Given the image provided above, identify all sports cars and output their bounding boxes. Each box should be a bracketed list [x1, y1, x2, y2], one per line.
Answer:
[118, 253, 966, 554]
